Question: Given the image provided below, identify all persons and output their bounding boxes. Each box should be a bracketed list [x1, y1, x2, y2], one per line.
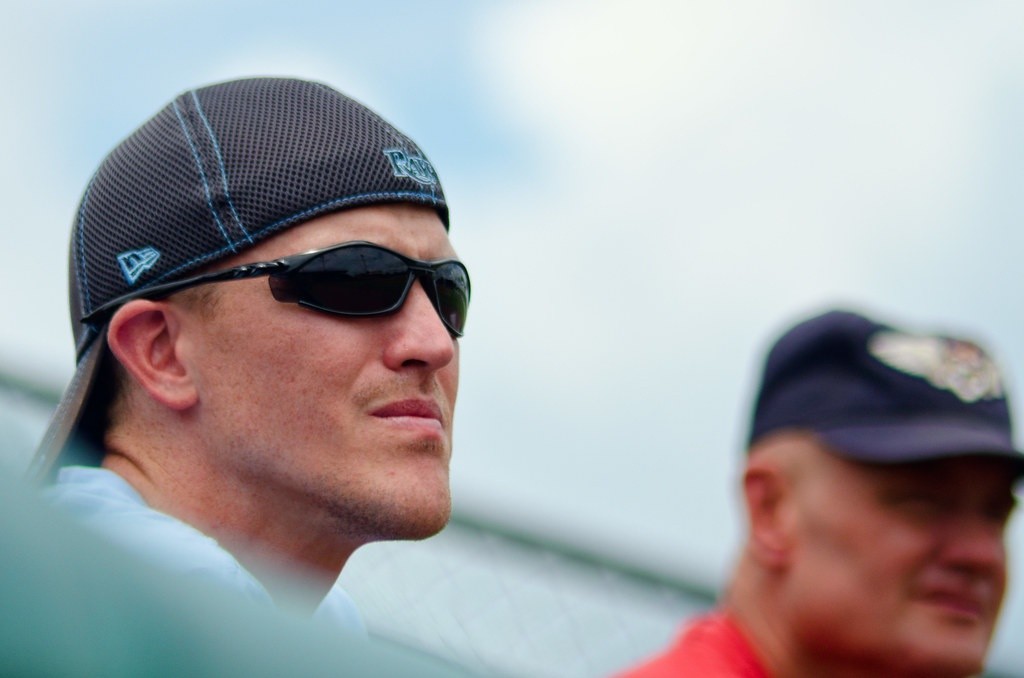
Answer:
[1, 74, 483, 675]
[600, 308, 1024, 678]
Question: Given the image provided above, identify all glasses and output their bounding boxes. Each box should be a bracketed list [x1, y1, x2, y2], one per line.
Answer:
[81, 242, 471, 341]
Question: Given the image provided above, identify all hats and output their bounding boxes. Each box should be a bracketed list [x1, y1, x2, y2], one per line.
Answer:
[32, 79, 450, 488]
[746, 310, 1024, 484]
